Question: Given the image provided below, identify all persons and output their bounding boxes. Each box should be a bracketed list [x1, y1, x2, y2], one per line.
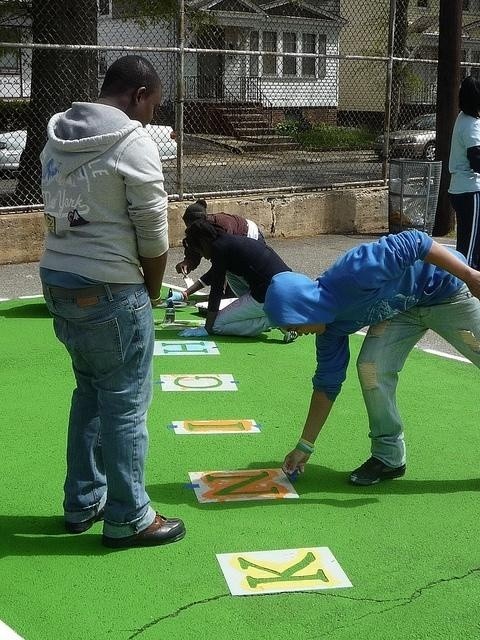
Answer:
[448, 74, 480, 272]
[166, 199, 266, 317]
[263, 230, 480, 487]
[37, 55, 188, 549]
[177, 220, 298, 343]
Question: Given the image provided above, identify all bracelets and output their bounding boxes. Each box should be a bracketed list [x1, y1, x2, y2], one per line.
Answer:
[297, 438, 314, 455]
[150, 295, 161, 302]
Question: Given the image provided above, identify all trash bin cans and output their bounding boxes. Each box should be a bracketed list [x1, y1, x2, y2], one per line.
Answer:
[388, 158, 442, 238]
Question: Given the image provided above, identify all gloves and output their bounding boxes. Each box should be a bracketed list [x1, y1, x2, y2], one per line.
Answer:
[166, 292, 185, 302]
[177, 326, 208, 336]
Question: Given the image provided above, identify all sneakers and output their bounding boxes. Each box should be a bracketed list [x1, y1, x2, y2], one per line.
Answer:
[279, 327, 298, 343]
[65, 509, 104, 534]
[103, 513, 186, 549]
[349, 457, 406, 485]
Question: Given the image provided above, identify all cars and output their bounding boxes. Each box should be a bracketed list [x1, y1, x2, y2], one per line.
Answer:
[1, 123, 181, 175]
[372, 112, 436, 163]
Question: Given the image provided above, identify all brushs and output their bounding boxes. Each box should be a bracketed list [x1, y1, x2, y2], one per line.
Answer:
[182, 266, 194, 288]
[164, 289, 176, 323]
